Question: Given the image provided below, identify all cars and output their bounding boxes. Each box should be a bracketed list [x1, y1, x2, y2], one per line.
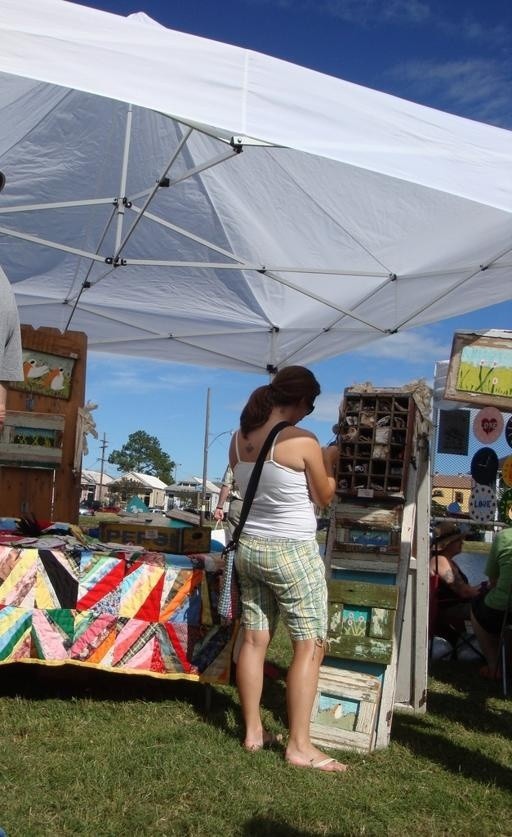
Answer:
[79, 500, 121, 516]
[149, 509, 166, 518]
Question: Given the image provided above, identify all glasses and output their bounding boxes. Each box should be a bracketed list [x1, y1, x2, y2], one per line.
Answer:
[305, 398, 315, 416]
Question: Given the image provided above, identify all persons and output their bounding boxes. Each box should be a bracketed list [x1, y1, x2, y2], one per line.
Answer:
[229, 367, 351, 773]
[0, 169, 25, 432]
[430, 521, 511, 680]
[214, 465, 243, 544]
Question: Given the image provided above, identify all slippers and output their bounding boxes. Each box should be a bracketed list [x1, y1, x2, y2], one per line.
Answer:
[312, 758, 347, 771]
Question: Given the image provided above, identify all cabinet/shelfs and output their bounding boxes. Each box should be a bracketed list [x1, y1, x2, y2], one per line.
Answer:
[335, 392, 415, 502]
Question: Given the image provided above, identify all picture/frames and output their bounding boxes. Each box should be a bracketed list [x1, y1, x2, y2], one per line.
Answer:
[8, 348, 77, 402]
[308, 666, 382, 756]
[321, 577, 398, 665]
[328, 503, 402, 574]
[0, 410, 65, 471]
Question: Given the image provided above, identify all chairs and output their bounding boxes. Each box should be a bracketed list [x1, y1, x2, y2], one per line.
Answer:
[429, 567, 488, 665]
[494, 584, 512, 697]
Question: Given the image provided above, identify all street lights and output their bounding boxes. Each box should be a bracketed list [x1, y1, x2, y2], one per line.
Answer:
[201, 431, 235, 526]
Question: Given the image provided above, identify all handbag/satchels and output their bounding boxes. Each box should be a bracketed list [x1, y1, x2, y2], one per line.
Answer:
[217, 543, 241, 619]
[210, 518, 225, 552]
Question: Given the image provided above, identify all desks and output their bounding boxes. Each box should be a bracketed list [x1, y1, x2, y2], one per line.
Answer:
[0, 541, 240, 711]
